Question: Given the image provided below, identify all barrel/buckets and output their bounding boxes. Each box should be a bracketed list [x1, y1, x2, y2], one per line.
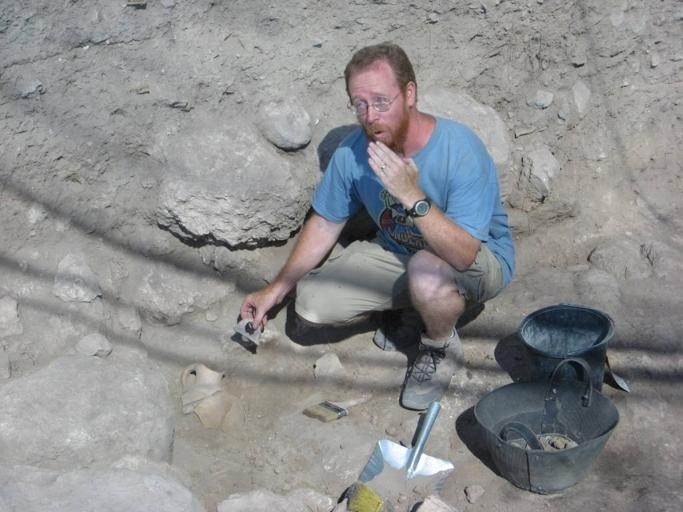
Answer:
[520, 304, 614, 392]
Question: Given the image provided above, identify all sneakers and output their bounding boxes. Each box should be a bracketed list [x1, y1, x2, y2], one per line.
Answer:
[373, 309, 425, 352]
[398, 325, 462, 410]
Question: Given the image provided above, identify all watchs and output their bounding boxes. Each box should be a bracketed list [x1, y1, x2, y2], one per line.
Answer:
[404, 194, 434, 219]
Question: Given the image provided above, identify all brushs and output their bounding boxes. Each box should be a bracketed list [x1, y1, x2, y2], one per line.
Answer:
[303, 393, 372, 422]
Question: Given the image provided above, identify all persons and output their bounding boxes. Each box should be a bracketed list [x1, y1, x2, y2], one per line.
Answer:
[237, 39, 517, 410]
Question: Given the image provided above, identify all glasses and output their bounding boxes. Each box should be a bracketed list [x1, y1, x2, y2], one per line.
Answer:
[344, 87, 405, 117]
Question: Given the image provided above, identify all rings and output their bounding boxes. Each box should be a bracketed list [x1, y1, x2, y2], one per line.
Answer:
[380, 164, 387, 170]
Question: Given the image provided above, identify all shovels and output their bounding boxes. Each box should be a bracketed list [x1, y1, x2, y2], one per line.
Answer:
[357, 402, 454, 512]
